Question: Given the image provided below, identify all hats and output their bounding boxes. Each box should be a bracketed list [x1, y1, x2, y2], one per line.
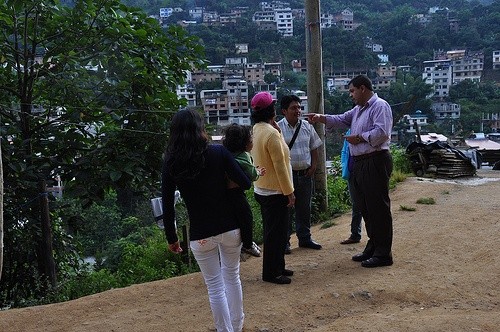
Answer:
[250, 92, 278, 112]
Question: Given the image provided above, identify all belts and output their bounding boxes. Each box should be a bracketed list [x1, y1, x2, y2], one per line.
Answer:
[293, 170, 308, 176]
[353, 153, 373, 160]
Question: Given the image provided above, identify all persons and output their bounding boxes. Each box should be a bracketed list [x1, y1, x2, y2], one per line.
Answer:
[303, 74, 393, 267]
[222, 123, 266, 256]
[161, 108, 251, 332]
[339, 129, 362, 244]
[277, 94, 323, 253]
[469, 131, 477, 139]
[247, 91, 296, 284]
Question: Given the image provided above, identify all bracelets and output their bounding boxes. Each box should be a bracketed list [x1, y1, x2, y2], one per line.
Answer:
[355, 134, 362, 143]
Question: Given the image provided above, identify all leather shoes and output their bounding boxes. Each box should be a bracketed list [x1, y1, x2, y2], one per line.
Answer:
[361, 257, 393, 268]
[353, 254, 368, 261]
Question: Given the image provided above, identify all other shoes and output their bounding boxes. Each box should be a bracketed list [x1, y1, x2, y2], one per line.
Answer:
[285, 247, 291, 254]
[299, 241, 321, 249]
[242, 245, 261, 257]
[263, 275, 291, 284]
[341, 238, 360, 244]
[281, 269, 293, 276]
